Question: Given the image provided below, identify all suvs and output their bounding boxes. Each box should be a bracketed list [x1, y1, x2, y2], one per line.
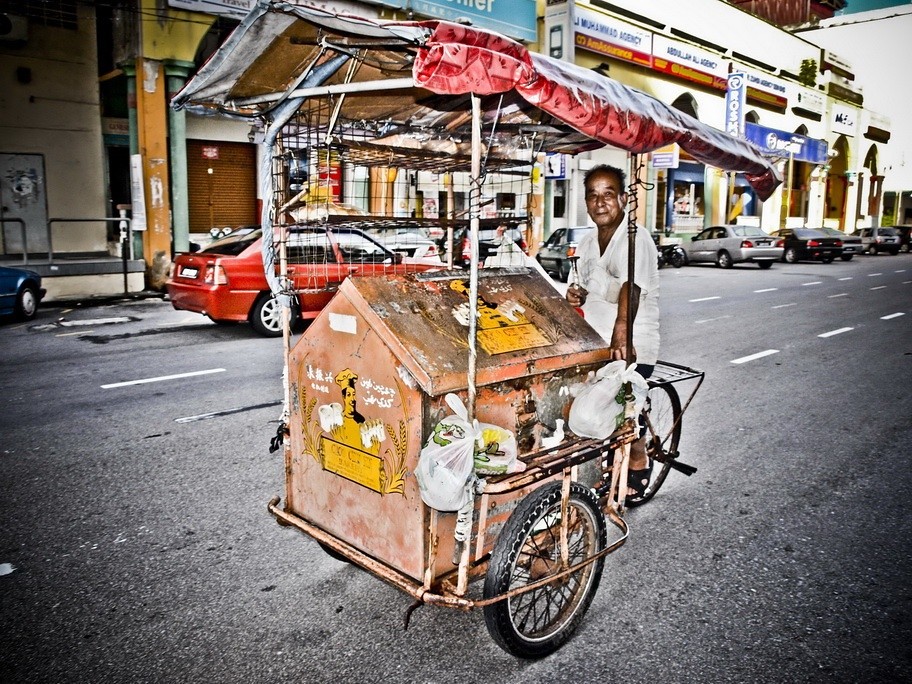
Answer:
[889, 224, 912, 255]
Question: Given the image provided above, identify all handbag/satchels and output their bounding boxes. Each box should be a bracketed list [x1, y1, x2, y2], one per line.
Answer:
[568, 359, 648, 443]
[415, 393, 485, 513]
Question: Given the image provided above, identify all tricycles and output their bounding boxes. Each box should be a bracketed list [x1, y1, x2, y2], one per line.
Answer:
[169, 0, 790, 659]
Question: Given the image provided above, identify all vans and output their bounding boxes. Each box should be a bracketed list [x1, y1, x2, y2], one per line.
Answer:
[850, 227, 901, 255]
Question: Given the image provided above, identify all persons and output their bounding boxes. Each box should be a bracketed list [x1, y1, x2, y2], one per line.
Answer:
[564, 165, 659, 505]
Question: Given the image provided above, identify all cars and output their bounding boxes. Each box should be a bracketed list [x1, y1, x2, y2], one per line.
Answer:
[681, 225, 785, 269]
[534, 226, 597, 282]
[166, 218, 530, 338]
[767, 227, 863, 264]
[0, 267, 47, 321]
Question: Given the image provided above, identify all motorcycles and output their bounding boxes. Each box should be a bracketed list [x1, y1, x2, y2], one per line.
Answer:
[651, 234, 688, 269]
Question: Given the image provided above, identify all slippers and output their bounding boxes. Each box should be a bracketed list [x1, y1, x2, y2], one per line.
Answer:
[623, 458, 655, 499]
